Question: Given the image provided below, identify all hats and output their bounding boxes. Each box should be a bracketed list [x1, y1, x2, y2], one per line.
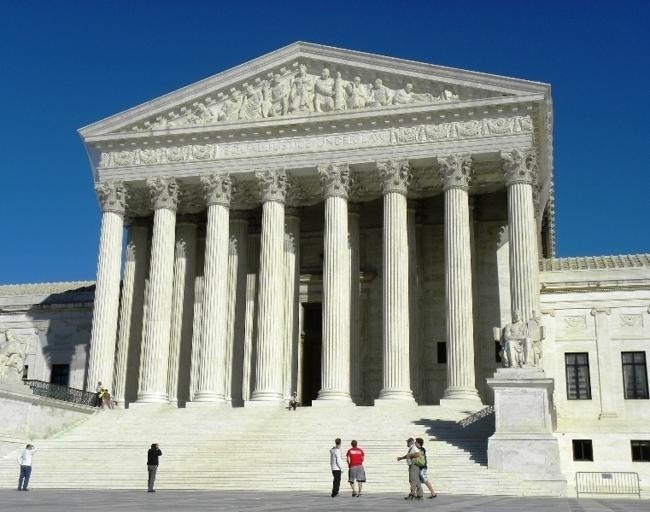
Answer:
[405, 438, 414, 444]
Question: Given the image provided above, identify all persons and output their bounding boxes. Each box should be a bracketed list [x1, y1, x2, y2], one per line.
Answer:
[289, 391, 299, 410]
[414, 438, 437, 498]
[503, 308, 544, 368]
[396, 438, 423, 500]
[346, 440, 366, 497]
[147, 444, 162, 491]
[95, 381, 114, 409]
[0, 329, 26, 373]
[183, 64, 459, 124]
[17, 444, 37, 490]
[330, 438, 343, 497]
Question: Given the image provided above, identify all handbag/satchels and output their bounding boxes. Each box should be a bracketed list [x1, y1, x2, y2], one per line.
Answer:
[408, 446, 426, 467]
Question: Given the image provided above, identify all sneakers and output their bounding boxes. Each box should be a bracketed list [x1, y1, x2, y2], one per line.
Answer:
[404, 493, 424, 501]
[428, 493, 437, 499]
